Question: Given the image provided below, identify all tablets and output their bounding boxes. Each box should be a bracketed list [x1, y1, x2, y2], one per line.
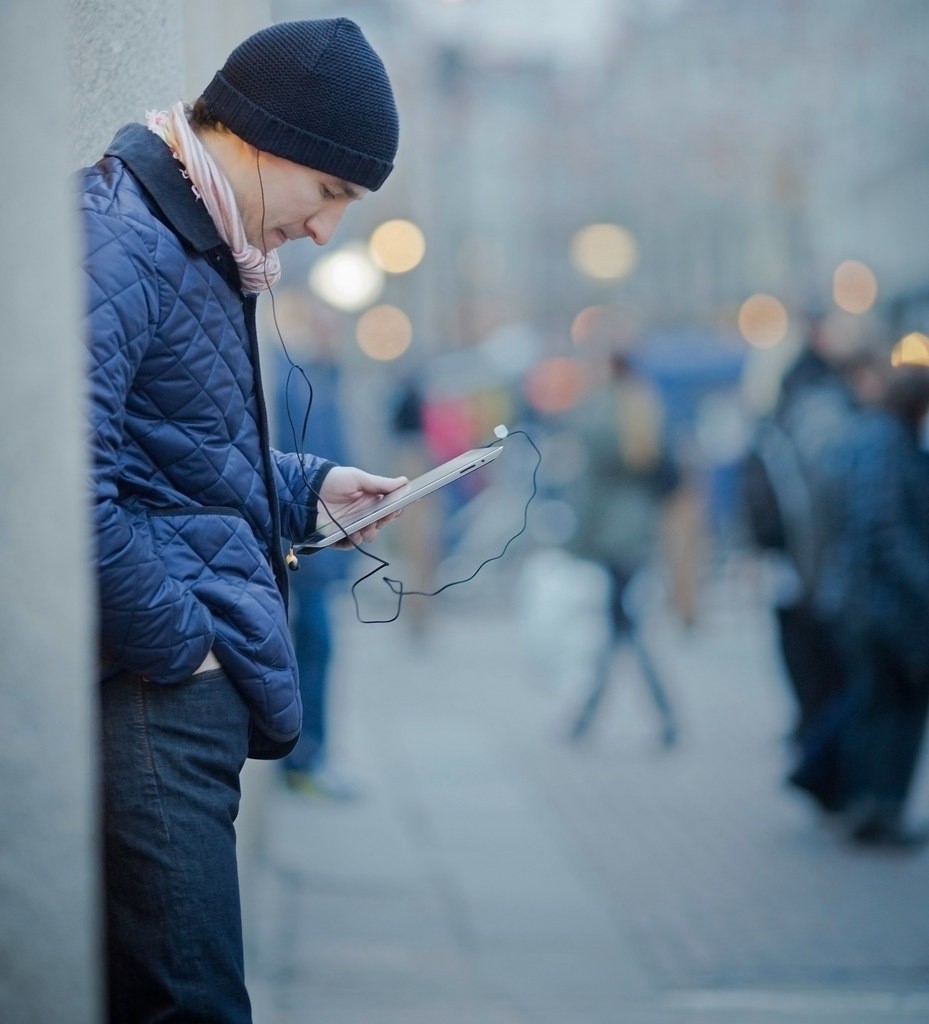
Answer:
[293, 445, 504, 555]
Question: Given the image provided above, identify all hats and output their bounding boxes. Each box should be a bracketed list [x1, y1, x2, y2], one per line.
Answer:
[202, 17, 399, 193]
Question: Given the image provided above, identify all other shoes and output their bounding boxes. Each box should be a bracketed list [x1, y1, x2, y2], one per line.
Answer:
[849, 801, 929, 851]
[787, 755, 851, 816]
[282, 760, 353, 801]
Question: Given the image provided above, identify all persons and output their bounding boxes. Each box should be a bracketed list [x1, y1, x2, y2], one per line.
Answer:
[562, 347, 693, 755]
[738, 308, 929, 842]
[270, 292, 363, 804]
[79, 14, 401, 1004]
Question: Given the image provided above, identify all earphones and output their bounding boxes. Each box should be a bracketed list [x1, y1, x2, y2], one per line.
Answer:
[287, 549, 299, 571]
